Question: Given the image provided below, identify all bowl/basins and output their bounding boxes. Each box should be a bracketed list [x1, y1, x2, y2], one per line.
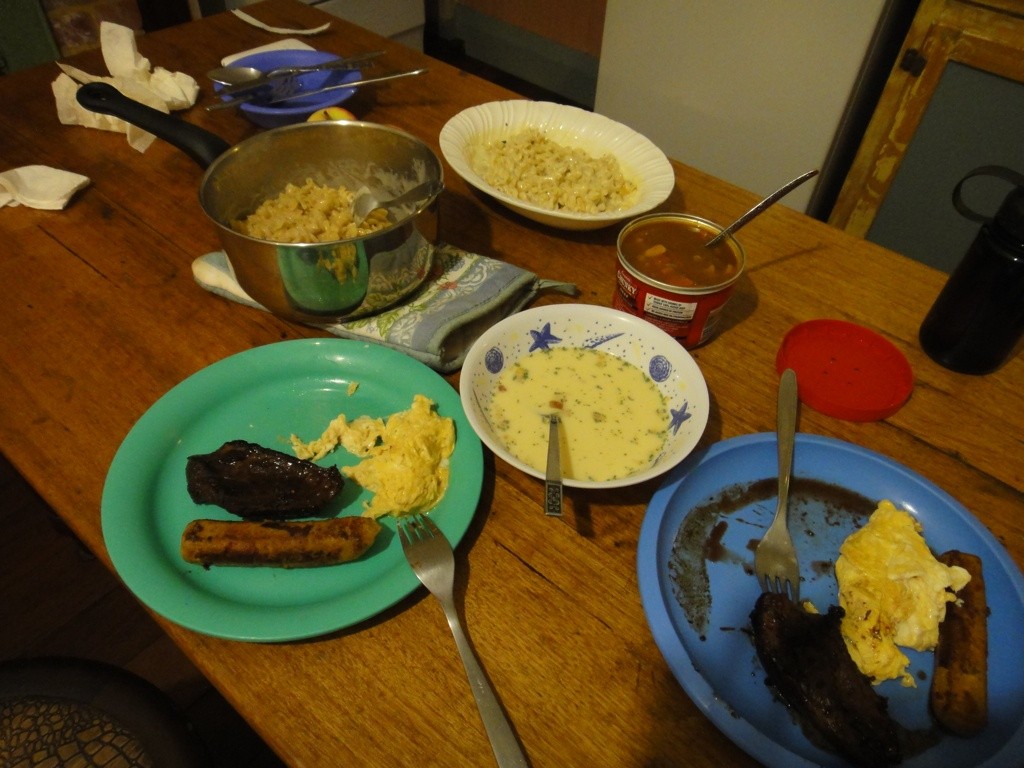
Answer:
[438, 98, 677, 230]
[215, 48, 364, 129]
[457, 301, 713, 492]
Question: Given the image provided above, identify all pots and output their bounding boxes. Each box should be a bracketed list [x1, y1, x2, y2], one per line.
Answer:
[73, 80, 443, 324]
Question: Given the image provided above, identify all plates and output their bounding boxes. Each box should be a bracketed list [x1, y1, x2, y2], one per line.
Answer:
[636, 427, 1024, 768]
[98, 339, 484, 644]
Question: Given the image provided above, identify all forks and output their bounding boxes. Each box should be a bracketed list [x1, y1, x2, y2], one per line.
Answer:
[394, 509, 529, 768]
[754, 367, 801, 603]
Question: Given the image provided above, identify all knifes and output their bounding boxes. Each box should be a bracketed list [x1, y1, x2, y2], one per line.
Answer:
[268, 66, 429, 104]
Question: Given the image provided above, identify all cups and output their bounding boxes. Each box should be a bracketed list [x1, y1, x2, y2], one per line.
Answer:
[918, 164, 1023, 378]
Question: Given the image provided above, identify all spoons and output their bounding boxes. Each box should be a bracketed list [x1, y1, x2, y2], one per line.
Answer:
[353, 177, 438, 225]
[210, 61, 372, 84]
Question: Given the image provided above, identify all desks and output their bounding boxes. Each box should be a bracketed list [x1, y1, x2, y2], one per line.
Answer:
[0, 2, 1024, 766]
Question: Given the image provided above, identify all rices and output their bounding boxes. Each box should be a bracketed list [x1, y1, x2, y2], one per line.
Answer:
[477, 129, 634, 217]
[234, 171, 399, 241]
